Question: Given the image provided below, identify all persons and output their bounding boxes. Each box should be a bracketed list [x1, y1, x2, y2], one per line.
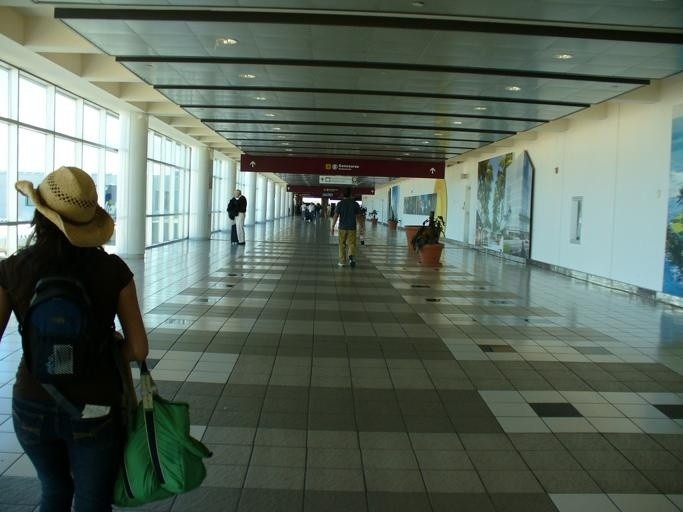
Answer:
[233, 190, 247, 245]
[0, 164, 151, 511]
[226, 193, 238, 245]
[293, 199, 338, 223]
[330, 187, 365, 268]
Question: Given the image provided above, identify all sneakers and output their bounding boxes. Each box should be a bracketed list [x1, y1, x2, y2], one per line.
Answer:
[338, 255, 356, 268]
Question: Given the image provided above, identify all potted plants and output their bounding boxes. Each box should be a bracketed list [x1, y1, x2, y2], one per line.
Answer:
[410, 216, 446, 267]
[361, 207, 367, 222]
[388, 209, 398, 230]
[369, 209, 378, 225]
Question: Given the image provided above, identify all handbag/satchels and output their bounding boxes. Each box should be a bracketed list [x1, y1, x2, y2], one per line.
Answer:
[114, 364, 213, 506]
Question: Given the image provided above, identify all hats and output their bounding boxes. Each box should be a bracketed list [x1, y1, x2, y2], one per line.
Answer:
[14, 166, 113, 247]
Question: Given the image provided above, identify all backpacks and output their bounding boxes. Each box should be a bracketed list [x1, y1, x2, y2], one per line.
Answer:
[19, 251, 111, 382]
